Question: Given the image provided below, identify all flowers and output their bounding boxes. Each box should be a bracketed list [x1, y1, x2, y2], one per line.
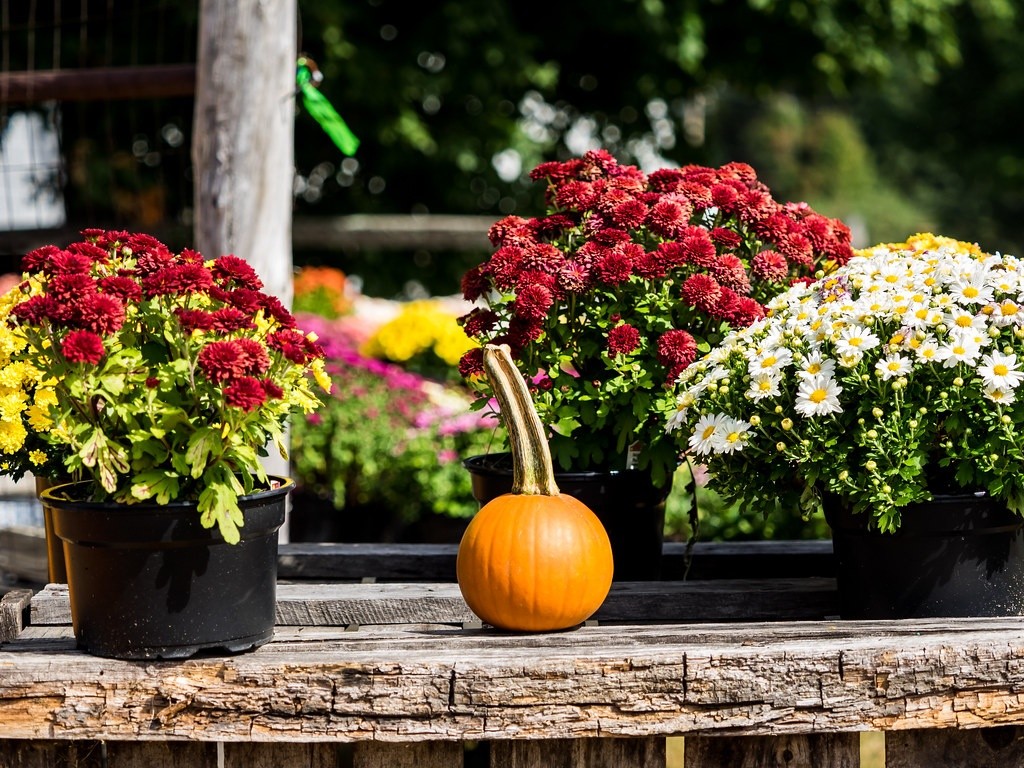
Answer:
[458, 151, 858, 475]
[1, 226, 334, 545]
[633, 231, 1023, 532]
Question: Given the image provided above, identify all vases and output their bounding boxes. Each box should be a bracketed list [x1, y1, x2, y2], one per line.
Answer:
[818, 481, 1024, 617]
[459, 451, 673, 580]
[35, 474, 76, 584]
[287, 486, 468, 546]
[40, 472, 295, 661]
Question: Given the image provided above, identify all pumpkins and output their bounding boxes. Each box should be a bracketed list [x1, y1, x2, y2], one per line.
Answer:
[456, 344, 613, 633]
